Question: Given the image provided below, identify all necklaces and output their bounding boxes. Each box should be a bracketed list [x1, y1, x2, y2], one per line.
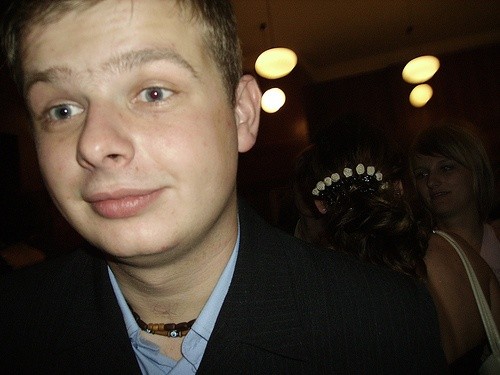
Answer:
[121, 294, 196, 337]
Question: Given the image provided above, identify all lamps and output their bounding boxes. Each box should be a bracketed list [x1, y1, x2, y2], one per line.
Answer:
[254, 0, 298, 114]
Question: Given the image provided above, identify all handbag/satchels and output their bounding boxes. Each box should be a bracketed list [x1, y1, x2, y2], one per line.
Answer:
[432, 230, 500, 375]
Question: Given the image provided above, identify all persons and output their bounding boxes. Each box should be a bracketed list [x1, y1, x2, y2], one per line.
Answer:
[408, 115, 500, 282]
[0, 0, 452, 375]
[288, 126, 500, 375]
